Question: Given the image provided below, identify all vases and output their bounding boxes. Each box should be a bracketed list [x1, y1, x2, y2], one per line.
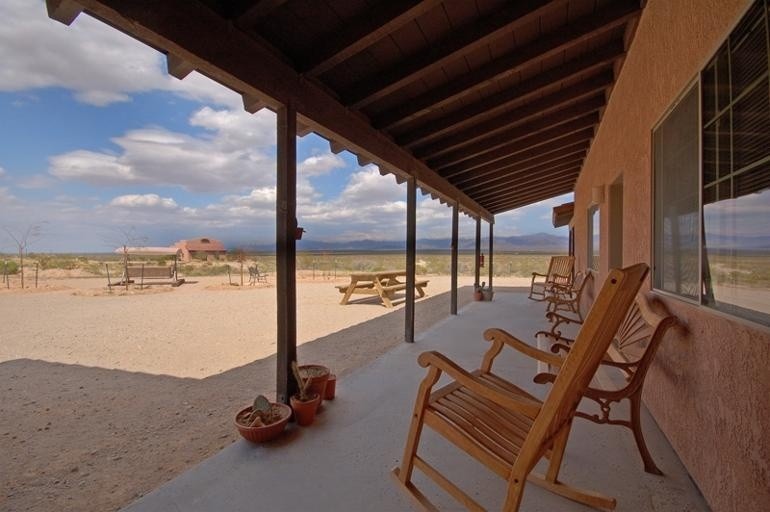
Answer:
[234, 366, 341, 443]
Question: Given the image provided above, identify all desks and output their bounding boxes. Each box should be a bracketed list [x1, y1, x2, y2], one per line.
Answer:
[331, 265, 432, 307]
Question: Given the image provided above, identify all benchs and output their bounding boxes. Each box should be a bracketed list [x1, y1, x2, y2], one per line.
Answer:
[335, 280, 429, 306]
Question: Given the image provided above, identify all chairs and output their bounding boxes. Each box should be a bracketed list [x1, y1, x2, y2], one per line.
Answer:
[528, 253, 680, 484]
[388, 262, 653, 512]
